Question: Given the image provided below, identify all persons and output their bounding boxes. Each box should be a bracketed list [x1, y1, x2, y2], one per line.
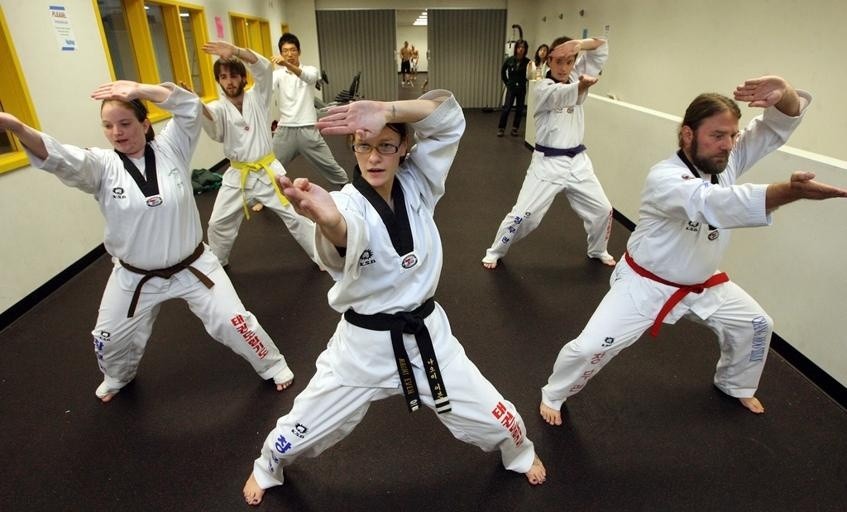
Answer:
[409, 43, 419, 81]
[481, 33, 616, 271]
[178, 40, 326, 273]
[0, 79, 295, 404]
[269, 32, 350, 189]
[495, 38, 530, 138]
[398, 41, 411, 85]
[538, 75, 847, 427]
[240, 87, 549, 507]
[524, 44, 550, 81]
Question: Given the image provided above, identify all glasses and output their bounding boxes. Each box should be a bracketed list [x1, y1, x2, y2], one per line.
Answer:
[352, 138, 404, 154]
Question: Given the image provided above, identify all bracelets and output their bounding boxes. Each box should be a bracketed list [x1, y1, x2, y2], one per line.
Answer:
[388, 100, 397, 124]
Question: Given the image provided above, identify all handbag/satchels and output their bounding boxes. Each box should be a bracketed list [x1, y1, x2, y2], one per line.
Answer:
[192, 168, 221, 192]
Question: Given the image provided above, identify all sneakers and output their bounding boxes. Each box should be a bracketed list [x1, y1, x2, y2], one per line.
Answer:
[498, 129, 518, 136]
